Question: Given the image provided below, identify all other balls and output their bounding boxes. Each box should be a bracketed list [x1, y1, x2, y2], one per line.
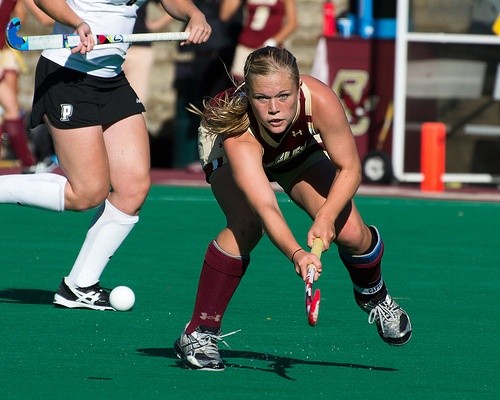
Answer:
[110, 286, 137, 311]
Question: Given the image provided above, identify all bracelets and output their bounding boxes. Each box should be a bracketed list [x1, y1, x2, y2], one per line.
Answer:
[291, 247, 303, 262]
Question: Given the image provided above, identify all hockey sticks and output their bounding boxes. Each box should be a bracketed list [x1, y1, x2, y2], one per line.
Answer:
[5, 17, 191, 53]
[305, 235, 322, 327]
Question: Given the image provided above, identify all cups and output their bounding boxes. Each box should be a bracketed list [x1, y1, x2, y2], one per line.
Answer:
[337, 0, 396, 38]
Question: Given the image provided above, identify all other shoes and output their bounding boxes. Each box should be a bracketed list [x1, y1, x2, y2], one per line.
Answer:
[23, 156, 57, 174]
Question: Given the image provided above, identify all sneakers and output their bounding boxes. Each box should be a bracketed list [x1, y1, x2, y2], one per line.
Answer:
[353, 284, 413, 346]
[54, 276, 113, 310]
[172, 321, 224, 371]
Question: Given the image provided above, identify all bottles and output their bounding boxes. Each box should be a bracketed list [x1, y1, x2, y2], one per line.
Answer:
[323, 0, 336, 37]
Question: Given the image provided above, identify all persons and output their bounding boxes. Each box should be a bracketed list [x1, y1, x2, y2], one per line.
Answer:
[175, 46, 413, 371]
[1, 0, 500, 173]
[0, 0, 211, 312]
